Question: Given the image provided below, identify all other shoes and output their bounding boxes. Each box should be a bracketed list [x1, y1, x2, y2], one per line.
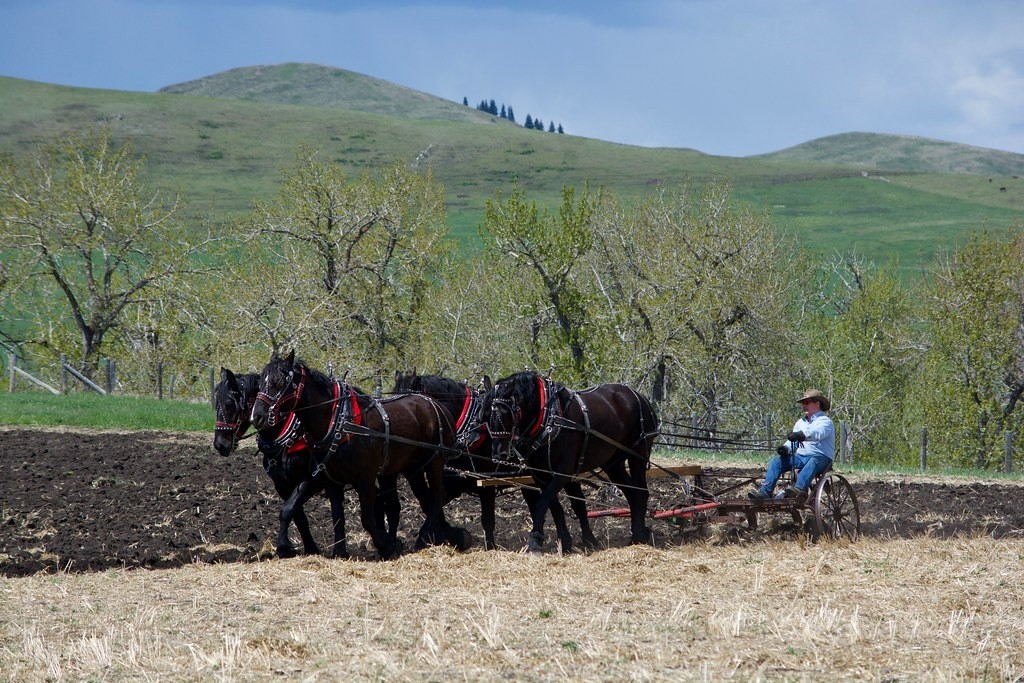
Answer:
[748, 489, 774, 508]
[786, 486, 808, 504]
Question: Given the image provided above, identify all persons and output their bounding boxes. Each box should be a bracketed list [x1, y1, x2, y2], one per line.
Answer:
[748, 390, 836, 502]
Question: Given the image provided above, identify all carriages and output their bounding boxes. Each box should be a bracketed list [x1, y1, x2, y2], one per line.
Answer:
[213, 349, 863, 564]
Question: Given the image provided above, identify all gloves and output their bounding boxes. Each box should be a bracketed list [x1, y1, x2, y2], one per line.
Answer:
[777, 445, 788, 457]
[787, 430, 805, 443]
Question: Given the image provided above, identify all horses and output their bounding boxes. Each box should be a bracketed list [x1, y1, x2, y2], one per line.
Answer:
[204, 346, 661, 560]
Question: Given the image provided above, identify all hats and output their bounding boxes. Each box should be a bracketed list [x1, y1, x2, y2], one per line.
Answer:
[795, 389, 830, 411]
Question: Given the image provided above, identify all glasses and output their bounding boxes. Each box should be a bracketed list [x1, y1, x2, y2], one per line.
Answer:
[801, 400, 816, 405]
